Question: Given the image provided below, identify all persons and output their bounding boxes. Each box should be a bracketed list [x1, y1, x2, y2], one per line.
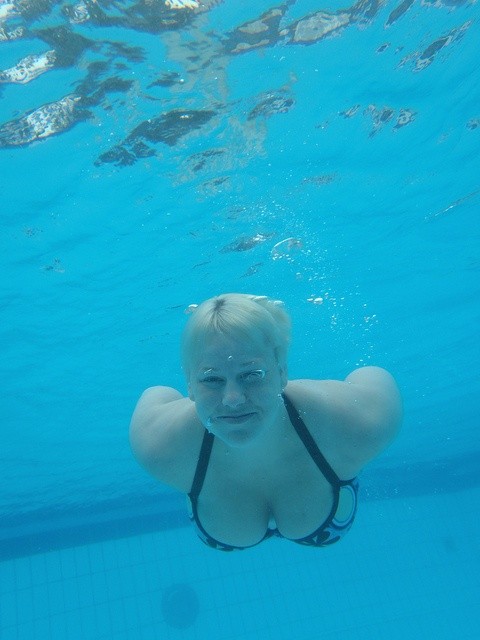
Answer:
[129, 293, 402, 553]
